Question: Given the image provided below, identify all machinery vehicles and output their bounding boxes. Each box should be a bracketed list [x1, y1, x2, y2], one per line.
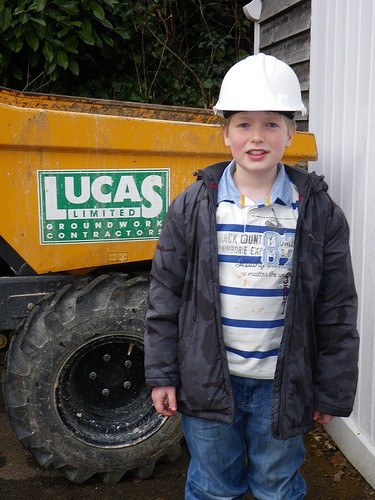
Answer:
[0, 84, 316, 478]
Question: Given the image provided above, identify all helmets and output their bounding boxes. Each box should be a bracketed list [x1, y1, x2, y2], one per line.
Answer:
[213, 53, 307, 117]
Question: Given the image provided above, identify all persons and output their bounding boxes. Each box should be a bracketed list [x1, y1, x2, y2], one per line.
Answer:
[144, 53, 361, 500]
